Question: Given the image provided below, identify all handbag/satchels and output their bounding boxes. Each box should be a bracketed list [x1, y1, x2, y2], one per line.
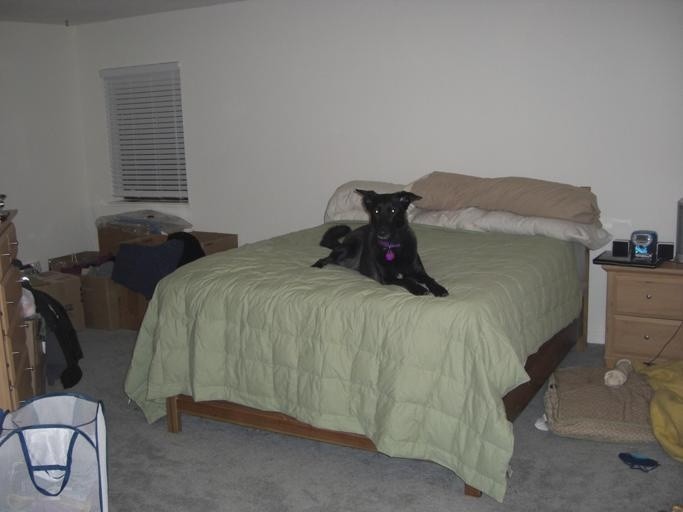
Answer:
[0, 390, 110, 512]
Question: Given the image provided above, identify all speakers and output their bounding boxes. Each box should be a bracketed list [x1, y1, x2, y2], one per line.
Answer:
[612, 239, 629, 257]
[656, 242, 677, 261]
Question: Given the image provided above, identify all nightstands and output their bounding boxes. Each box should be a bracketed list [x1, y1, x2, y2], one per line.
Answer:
[601, 264, 683, 372]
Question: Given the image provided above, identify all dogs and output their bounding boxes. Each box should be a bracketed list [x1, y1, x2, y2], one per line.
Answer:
[310, 188, 449, 298]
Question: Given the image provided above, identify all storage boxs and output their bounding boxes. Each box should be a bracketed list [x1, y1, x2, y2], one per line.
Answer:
[25, 210, 239, 331]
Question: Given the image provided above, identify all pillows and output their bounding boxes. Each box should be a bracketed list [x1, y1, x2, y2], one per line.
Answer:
[412, 206, 613, 250]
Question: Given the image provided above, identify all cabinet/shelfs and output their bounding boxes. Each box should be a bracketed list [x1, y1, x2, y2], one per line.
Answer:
[0, 208, 48, 413]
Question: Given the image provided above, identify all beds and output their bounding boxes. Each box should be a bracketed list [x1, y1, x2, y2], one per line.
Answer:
[153, 187, 596, 498]
